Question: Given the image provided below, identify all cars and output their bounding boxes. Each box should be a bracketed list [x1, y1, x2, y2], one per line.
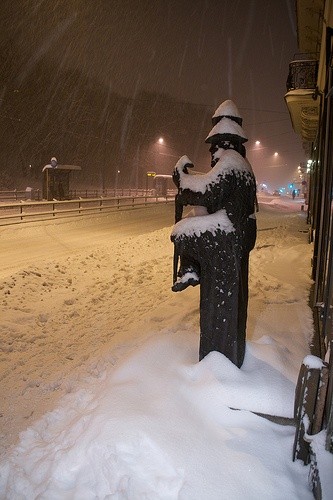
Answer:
[273, 190, 281, 196]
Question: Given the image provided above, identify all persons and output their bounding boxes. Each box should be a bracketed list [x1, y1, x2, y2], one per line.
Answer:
[292, 190, 295, 201]
[171, 117, 257, 294]
